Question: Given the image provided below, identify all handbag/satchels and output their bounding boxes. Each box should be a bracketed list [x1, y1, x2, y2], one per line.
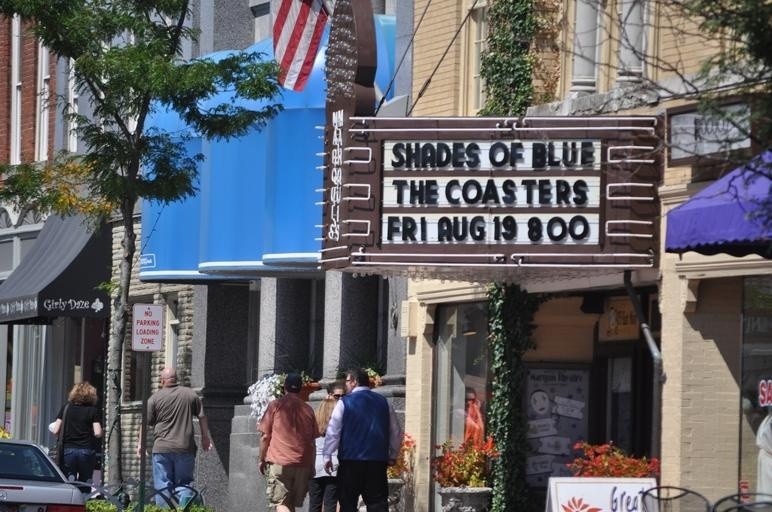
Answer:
[56, 441, 64, 466]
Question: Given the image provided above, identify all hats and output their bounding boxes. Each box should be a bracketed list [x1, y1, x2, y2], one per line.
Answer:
[285, 372, 303, 393]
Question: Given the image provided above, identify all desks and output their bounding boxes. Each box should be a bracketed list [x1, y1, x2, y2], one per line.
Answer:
[437, 486, 493, 512]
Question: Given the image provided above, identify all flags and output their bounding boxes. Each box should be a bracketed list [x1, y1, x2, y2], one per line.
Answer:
[266, 0, 330, 95]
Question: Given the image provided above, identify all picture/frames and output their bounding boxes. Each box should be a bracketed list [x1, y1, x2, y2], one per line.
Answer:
[598, 293, 661, 341]
[516, 359, 594, 491]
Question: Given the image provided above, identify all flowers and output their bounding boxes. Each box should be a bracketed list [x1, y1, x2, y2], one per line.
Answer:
[565, 440, 661, 481]
[244, 373, 287, 428]
[385, 431, 414, 482]
[430, 437, 500, 487]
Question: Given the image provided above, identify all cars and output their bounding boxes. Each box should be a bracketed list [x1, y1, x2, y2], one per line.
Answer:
[1, 439, 86, 511]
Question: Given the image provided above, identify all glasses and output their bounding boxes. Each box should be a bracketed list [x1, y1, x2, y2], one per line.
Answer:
[332, 393, 345, 399]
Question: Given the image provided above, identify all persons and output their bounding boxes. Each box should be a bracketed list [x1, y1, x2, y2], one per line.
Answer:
[135, 365, 212, 512]
[326, 380, 346, 400]
[321, 366, 404, 511]
[309, 395, 343, 511]
[46, 381, 102, 499]
[257, 371, 319, 511]
